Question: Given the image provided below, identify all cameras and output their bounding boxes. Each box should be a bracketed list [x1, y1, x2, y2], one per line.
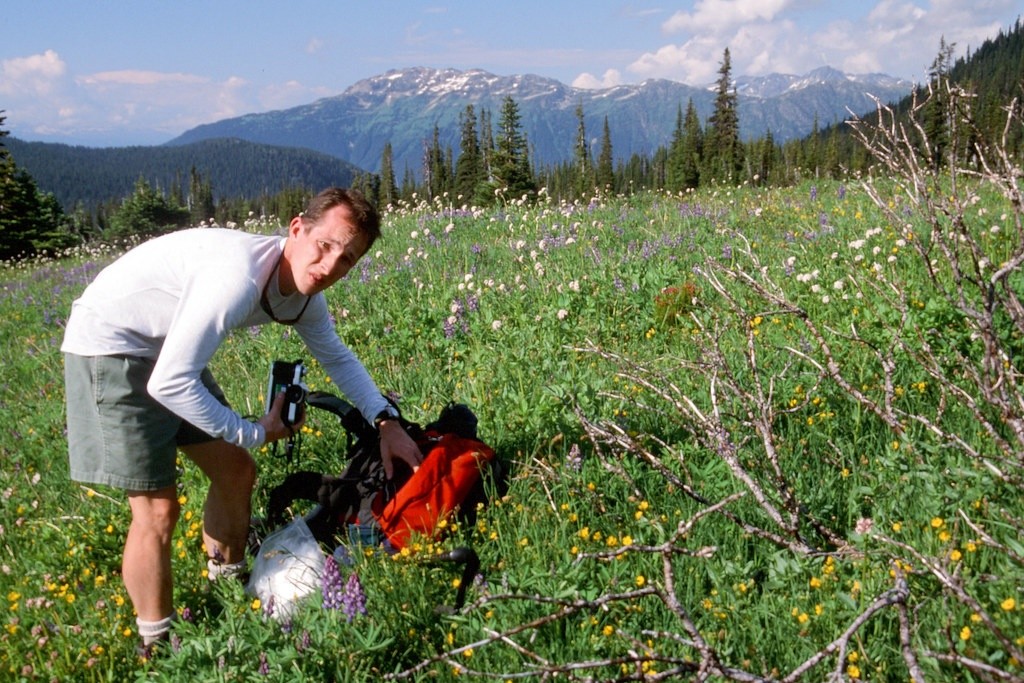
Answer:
[265, 359, 306, 425]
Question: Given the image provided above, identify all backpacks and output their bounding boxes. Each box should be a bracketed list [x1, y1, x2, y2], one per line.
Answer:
[380, 433, 493, 555]
[263, 387, 434, 556]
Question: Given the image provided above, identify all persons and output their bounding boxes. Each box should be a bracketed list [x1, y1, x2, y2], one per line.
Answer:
[58, 186, 425, 680]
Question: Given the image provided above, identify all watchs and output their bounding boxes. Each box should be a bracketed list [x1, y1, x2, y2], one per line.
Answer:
[373, 403, 399, 432]
